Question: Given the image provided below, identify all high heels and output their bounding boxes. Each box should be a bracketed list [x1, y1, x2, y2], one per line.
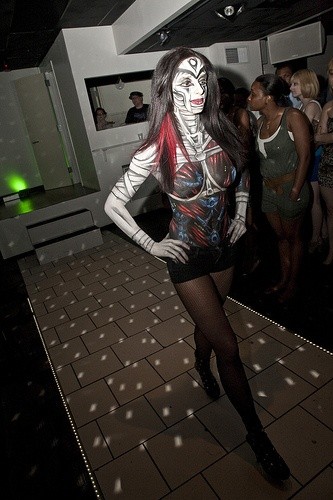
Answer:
[245, 432, 291, 481]
[194, 361, 221, 400]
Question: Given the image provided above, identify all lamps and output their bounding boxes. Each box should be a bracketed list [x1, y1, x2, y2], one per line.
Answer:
[215, 4, 245, 22]
[160, 31, 170, 47]
[117, 79, 123, 90]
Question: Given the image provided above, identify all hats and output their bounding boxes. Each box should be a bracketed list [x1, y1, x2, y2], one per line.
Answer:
[129, 91, 143, 100]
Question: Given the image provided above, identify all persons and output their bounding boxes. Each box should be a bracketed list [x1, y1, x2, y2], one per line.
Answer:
[125, 91, 151, 125]
[104, 47, 291, 481]
[212, 58, 333, 292]
[94, 108, 113, 131]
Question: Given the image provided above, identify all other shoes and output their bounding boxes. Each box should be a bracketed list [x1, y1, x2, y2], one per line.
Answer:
[264, 285, 280, 296]
[278, 292, 295, 303]
